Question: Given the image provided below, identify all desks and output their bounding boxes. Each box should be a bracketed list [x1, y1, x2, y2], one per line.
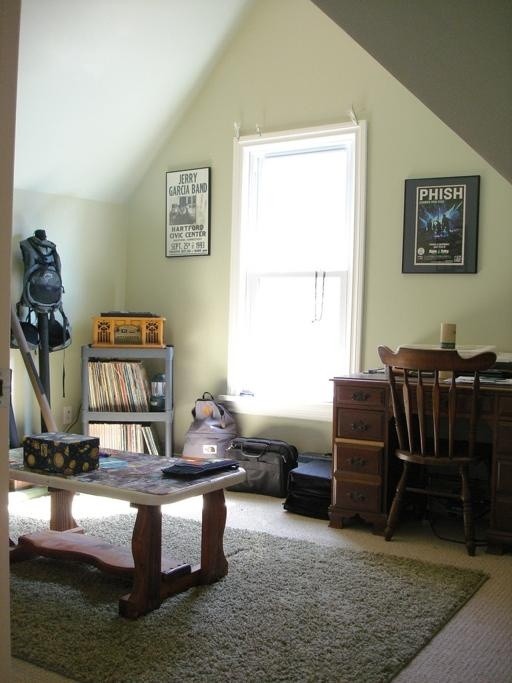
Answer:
[329, 372, 510, 557]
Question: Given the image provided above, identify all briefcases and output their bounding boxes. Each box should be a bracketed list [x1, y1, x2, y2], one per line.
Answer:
[296, 451, 333, 467]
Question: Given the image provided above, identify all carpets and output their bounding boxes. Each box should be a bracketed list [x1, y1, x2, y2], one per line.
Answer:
[11, 509, 489, 682]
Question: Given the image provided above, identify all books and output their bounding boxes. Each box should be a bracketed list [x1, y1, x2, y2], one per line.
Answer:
[89, 422, 161, 456]
[88, 360, 153, 412]
[444, 376, 512, 386]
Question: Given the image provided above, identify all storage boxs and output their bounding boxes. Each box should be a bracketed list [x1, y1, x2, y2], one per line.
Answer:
[23, 433, 97, 476]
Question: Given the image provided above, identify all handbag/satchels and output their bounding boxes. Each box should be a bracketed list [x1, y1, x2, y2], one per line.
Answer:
[181, 391, 236, 459]
[224, 437, 298, 498]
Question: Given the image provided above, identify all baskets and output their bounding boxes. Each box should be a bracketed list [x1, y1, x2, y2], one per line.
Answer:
[93, 317, 165, 349]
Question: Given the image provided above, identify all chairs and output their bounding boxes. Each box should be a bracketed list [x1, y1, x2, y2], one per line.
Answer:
[378, 341, 497, 557]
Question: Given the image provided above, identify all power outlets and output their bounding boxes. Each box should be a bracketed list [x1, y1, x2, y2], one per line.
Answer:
[62, 405, 73, 424]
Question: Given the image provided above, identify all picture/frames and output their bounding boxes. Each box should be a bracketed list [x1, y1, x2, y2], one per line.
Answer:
[402, 177, 479, 272]
[163, 167, 212, 257]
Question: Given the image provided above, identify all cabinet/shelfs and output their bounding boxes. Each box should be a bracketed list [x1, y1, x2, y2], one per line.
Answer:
[79, 343, 176, 459]
